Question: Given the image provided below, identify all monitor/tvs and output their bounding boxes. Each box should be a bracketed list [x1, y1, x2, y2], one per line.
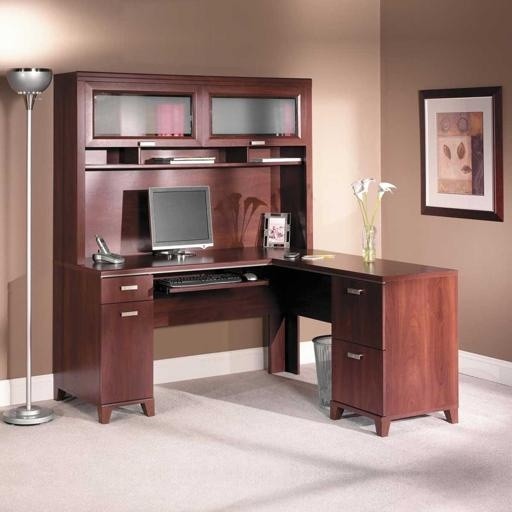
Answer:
[149, 186, 215, 255]
[151, 254, 214, 267]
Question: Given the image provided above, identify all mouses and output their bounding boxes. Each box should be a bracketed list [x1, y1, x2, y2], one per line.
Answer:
[240, 272, 258, 282]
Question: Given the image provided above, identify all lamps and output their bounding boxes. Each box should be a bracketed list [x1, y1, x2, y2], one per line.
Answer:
[3, 67, 52, 426]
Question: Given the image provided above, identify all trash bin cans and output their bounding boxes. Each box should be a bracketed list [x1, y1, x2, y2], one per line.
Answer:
[312, 335, 333, 405]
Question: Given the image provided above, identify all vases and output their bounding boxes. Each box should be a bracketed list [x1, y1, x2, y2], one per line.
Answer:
[361, 225, 377, 262]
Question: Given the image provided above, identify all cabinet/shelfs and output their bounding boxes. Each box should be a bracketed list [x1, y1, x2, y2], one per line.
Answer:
[52, 72, 313, 266]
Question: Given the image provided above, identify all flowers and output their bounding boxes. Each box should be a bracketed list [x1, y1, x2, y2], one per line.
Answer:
[351, 177, 398, 262]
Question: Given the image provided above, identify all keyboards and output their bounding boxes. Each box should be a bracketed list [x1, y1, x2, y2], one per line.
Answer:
[156, 272, 242, 287]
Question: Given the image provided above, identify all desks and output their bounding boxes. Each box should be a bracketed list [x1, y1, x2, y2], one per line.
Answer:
[53, 246, 458, 436]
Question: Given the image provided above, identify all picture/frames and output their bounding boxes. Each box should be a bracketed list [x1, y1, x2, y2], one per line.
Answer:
[418, 86, 503, 222]
[263, 213, 292, 248]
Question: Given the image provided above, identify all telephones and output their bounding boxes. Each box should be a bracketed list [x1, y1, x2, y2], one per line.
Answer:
[92, 234, 126, 264]
